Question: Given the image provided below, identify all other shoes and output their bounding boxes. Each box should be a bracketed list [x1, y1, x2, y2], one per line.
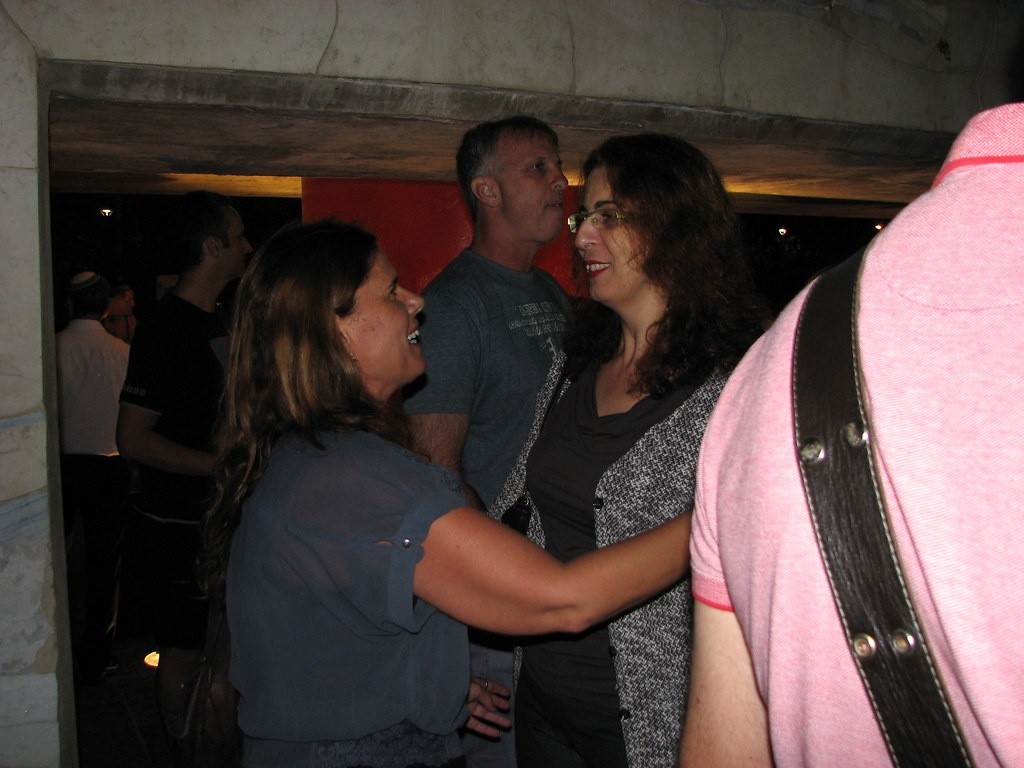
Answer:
[79, 655, 121, 692]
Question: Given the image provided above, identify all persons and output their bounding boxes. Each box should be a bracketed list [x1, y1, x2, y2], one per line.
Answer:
[402, 114, 588, 768]
[196, 217, 693, 768]
[679, 26, 1023, 768]
[53, 189, 255, 768]
[487, 133, 784, 768]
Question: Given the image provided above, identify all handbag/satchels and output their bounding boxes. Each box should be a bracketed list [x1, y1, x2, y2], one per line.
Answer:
[179, 562, 240, 768]
[466, 367, 568, 652]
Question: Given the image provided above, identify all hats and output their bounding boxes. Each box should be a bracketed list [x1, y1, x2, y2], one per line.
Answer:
[70, 271, 100, 291]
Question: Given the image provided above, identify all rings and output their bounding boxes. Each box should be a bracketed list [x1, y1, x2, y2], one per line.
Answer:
[483, 680, 488, 690]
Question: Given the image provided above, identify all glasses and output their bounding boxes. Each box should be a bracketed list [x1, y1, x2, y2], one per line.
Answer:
[567, 209, 642, 233]
